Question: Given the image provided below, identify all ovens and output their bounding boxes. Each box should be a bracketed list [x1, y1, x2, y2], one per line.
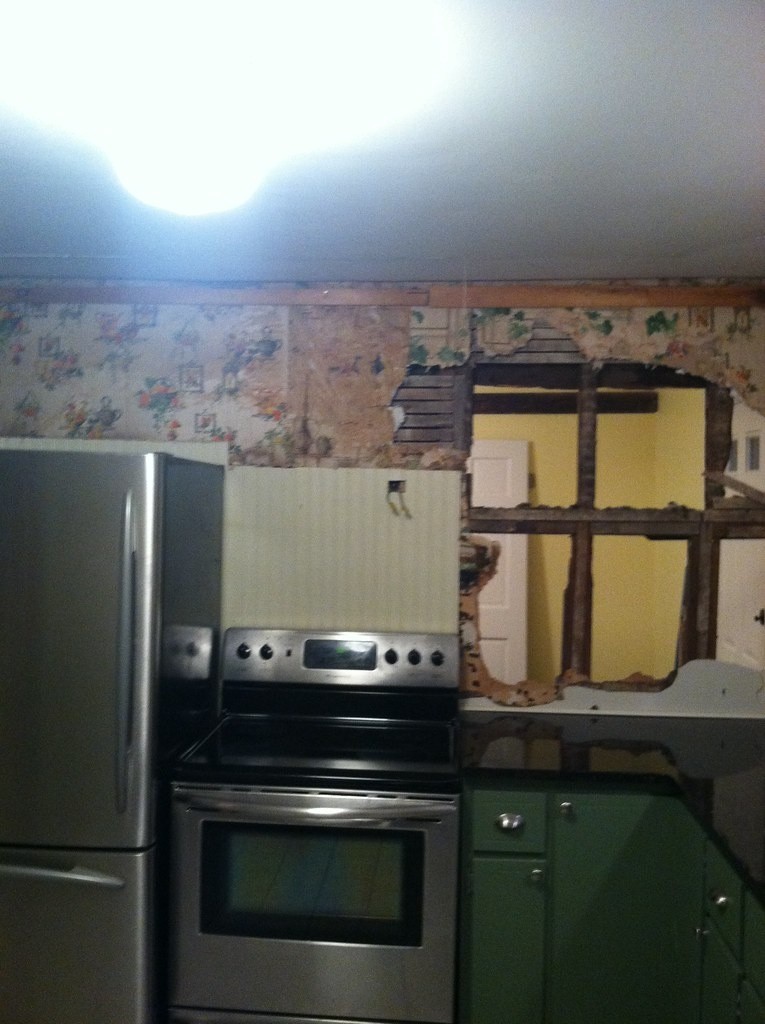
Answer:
[165, 627, 457, 1024]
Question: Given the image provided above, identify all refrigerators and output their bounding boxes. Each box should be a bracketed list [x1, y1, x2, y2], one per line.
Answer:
[0, 452, 225, 1024]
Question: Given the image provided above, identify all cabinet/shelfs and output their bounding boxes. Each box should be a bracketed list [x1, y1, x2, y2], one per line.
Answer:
[459, 775, 765, 1024]
